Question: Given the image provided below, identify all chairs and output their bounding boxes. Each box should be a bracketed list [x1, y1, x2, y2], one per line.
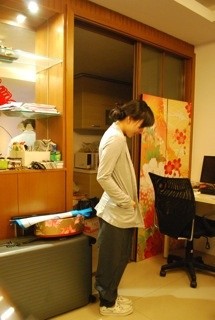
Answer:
[148, 172, 215, 288]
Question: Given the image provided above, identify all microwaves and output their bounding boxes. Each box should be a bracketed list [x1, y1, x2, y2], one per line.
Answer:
[75, 153, 99, 169]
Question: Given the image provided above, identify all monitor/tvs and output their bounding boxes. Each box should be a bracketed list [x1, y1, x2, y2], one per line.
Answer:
[200, 156, 215, 184]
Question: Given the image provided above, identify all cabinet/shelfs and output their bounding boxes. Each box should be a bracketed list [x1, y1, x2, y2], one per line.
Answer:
[74, 167, 105, 201]
[0, 168, 66, 242]
[73, 73, 133, 131]
[0, 45, 66, 169]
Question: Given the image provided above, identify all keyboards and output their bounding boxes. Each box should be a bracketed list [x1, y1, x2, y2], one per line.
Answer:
[201, 188, 215, 195]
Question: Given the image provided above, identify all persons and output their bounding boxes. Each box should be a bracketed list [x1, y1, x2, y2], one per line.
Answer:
[94, 98, 155, 316]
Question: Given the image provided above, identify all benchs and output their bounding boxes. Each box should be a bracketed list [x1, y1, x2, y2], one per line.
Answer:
[0, 235, 93, 320]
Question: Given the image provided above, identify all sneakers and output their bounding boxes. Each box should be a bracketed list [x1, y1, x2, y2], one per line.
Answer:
[100, 301, 134, 316]
[115, 296, 134, 305]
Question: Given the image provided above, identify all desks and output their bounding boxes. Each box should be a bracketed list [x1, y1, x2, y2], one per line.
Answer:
[163, 189, 215, 258]
